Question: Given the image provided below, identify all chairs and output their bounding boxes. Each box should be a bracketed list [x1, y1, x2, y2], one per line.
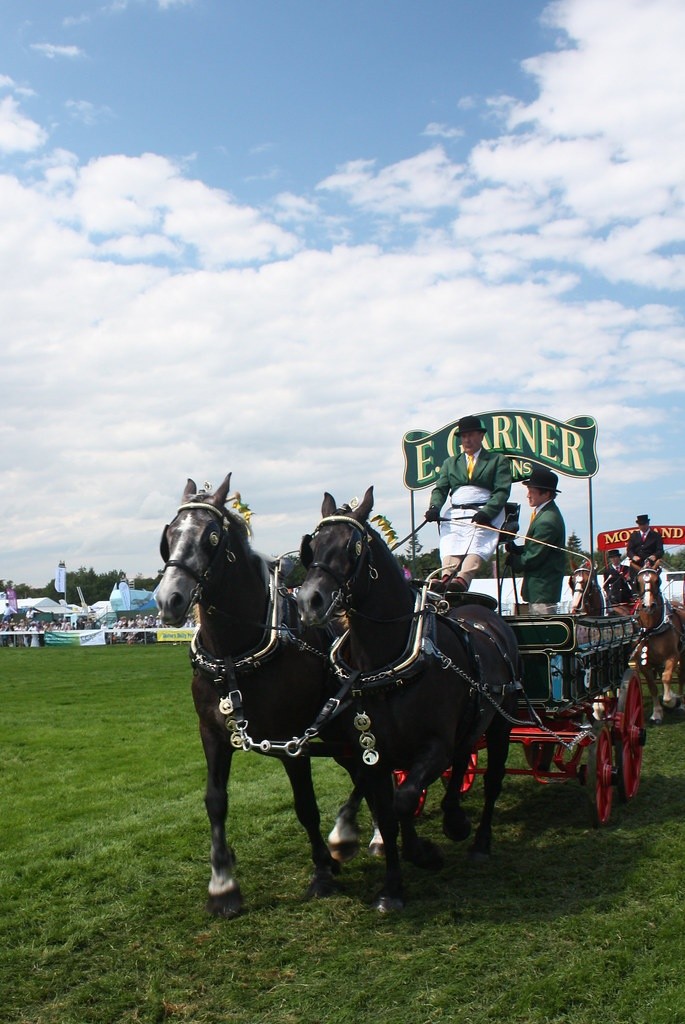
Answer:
[494, 502, 524, 616]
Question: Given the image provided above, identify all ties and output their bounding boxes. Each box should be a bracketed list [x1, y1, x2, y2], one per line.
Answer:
[530, 509, 536, 526]
[616, 566, 619, 573]
[642, 532, 645, 543]
[467, 454, 475, 480]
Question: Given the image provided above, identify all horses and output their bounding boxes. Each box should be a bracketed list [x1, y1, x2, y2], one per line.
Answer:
[568, 557, 634, 721]
[151, 472, 388, 917]
[292, 483, 520, 916]
[629, 558, 684, 726]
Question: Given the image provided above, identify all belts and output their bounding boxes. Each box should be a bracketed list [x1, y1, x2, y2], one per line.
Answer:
[451, 503, 486, 511]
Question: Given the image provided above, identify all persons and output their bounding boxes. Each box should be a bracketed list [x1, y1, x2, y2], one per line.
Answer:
[402, 564, 410, 579]
[424, 416, 512, 594]
[0, 600, 195, 648]
[505, 468, 567, 614]
[603, 550, 630, 583]
[579, 551, 598, 572]
[627, 514, 665, 590]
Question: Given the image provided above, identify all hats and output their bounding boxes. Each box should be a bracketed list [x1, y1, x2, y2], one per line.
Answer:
[636, 515, 651, 524]
[522, 469, 561, 493]
[607, 550, 621, 558]
[454, 416, 488, 437]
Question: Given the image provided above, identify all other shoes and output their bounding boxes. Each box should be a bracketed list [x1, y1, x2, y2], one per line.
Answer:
[430, 574, 469, 593]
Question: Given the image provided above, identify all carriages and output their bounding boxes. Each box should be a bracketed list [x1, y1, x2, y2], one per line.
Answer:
[154, 470, 685, 920]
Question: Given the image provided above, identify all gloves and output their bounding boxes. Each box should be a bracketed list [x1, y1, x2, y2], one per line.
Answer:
[425, 504, 440, 522]
[505, 541, 525, 574]
[471, 511, 491, 525]
